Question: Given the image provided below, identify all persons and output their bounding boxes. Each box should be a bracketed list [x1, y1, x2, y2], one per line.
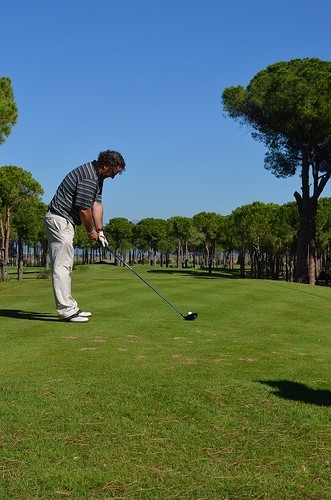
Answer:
[44, 149, 126, 322]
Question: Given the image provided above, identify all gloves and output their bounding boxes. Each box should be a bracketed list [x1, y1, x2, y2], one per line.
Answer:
[98, 231, 108, 248]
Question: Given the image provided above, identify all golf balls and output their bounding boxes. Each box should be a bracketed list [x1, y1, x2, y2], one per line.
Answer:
[187, 311, 192, 315]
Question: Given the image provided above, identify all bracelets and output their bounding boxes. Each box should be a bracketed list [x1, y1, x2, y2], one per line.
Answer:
[87, 229, 94, 234]
[96, 227, 104, 232]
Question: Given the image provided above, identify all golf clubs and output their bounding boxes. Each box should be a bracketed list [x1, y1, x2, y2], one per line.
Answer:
[98, 239, 198, 321]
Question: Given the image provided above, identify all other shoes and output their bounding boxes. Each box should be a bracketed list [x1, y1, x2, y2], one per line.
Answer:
[63, 314, 89, 322]
[75, 310, 92, 316]
